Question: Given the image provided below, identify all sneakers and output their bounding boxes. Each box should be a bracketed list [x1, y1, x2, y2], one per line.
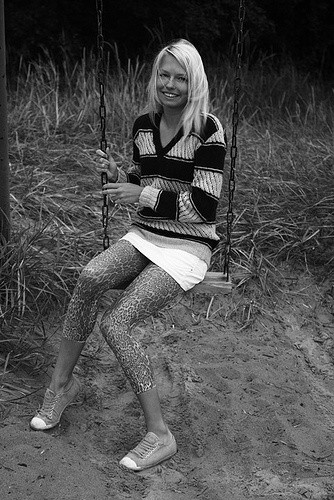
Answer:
[30, 374, 81, 429]
[119, 430, 178, 470]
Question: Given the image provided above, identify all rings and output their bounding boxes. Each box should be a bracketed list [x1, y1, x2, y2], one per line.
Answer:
[100, 158, 103, 163]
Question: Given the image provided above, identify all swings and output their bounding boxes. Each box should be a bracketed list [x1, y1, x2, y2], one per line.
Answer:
[92, 0, 247, 294]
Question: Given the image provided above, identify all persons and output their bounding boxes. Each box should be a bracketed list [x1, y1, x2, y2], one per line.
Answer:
[29, 39, 226, 472]
